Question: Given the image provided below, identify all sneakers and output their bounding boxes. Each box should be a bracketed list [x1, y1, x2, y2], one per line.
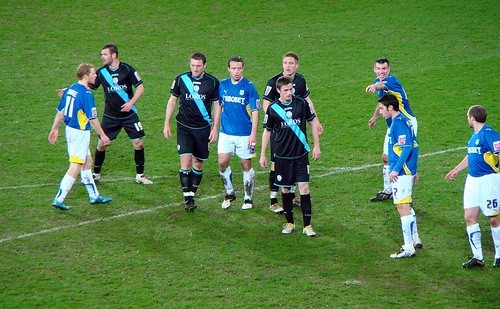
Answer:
[492, 258, 500, 267]
[52, 199, 72, 210]
[389, 249, 416, 258]
[270, 202, 284, 213]
[462, 257, 485, 268]
[185, 199, 193, 212]
[136, 174, 153, 185]
[192, 200, 197, 209]
[222, 194, 235, 209]
[81, 173, 101, 183]
[292, 198, 301, 207]
[282, 222, 295, 233]
[370, 191, 392, 202]
[303, 225, 316, 236]
[402, 237, 423, 248]
[90, 195, 112, 204]
[242, 200, 253, 210]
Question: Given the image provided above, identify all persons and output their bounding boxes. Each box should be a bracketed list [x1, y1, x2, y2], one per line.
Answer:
[218, 56, 259, 209]
[445, 105, 500, 268]
[48, 62, 113, 210]
[259, 76, 322, 236]
[365, 58, 418, 204]
[163, 52, 220, 211]
[54, 44, 155, 184]
[378, 92, 423, 259]
[263, 51, 324, 212]
